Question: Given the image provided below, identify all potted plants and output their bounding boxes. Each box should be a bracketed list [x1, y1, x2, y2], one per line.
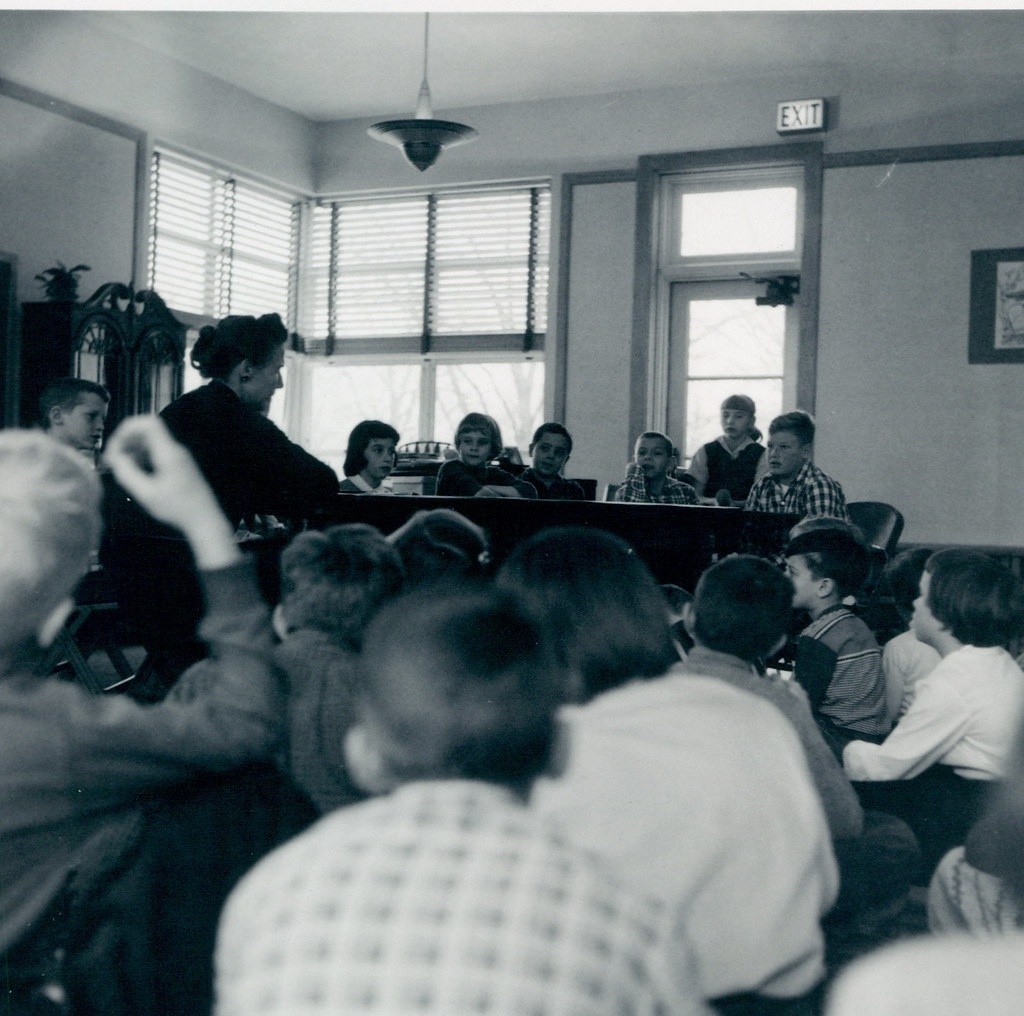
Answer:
[33, 261, 93, 301]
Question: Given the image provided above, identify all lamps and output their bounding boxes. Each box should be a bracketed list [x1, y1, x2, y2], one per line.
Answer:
[365, 13, 478, 172]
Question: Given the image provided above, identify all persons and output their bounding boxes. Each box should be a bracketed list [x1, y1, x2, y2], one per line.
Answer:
[747, 412, 851, 564]
[0, 413, 285, 1016]
[523, 422, 584, 498]
[615, 431, 702, 503]
[690, 395, 768, 507]
[213, 591, 704, 1016]
[162, 509, 1023, 1016]
[436, 413, 537, 498]
[340, 421, 400, 491]
[31, 381, 110, 462]
[125, 311, 339, 669]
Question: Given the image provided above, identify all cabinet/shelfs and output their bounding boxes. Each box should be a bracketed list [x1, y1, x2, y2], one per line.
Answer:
[17, 283, 193, 451]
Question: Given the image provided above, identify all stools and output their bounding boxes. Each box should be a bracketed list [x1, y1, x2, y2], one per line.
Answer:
[38, 603, 137, 697]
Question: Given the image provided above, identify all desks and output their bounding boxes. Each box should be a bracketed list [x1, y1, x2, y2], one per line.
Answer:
[335, 491, 802, 597]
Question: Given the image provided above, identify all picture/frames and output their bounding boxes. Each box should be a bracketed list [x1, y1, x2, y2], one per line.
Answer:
[969, 247, 1024, 365]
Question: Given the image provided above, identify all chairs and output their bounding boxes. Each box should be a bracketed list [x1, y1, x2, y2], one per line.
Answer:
[846, 501, 904, 560]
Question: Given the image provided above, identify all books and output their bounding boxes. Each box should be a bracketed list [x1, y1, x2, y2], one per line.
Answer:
[393, 476, 436, 494]
